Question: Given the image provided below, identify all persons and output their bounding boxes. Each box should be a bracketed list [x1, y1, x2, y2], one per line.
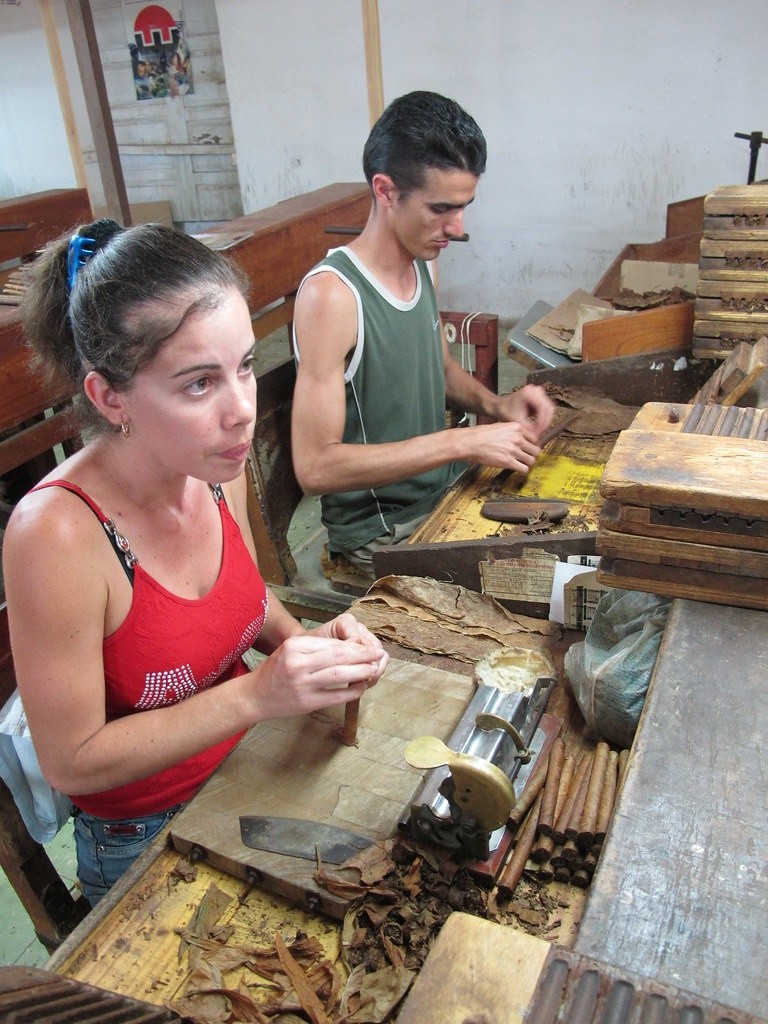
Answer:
[135, 51, 191, 96]
[0, 217, 391, 909]
[290, 90, 557, 580]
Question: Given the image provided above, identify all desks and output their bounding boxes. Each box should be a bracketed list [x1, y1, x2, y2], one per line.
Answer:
[42, 371, 642, 1024]
[0, 182, 375, 481]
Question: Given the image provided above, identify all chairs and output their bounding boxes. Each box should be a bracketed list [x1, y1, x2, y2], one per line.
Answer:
[0, 603, 92, 957]
[243, 353, 376, 627]
